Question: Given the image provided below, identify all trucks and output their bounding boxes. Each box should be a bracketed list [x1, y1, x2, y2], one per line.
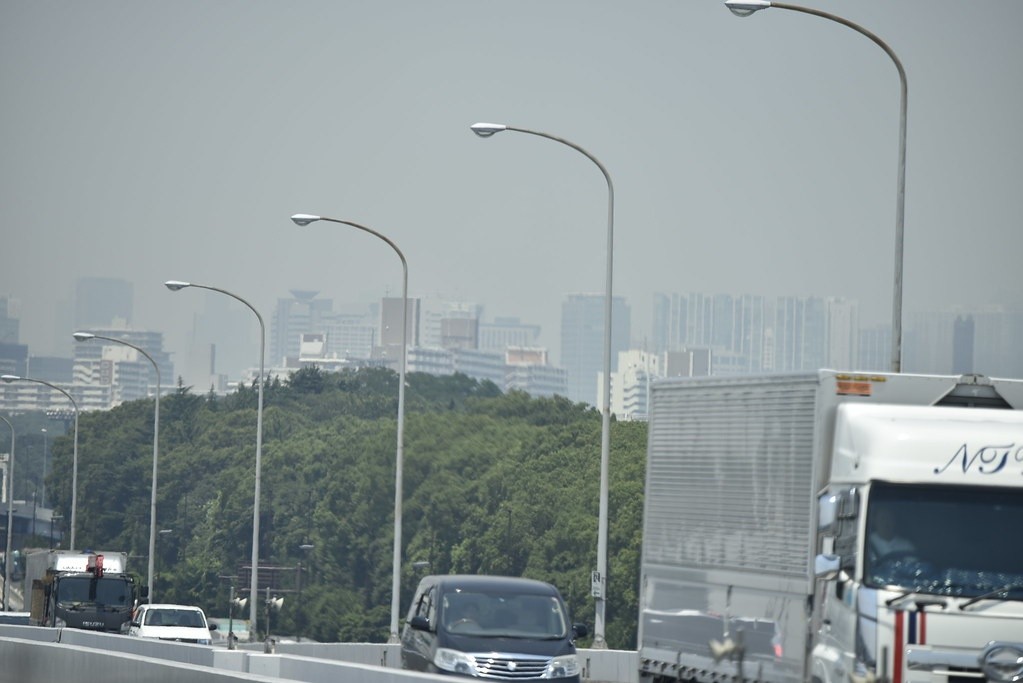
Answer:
[24, 549, 140, 634]
[637, 369, 1023, 683]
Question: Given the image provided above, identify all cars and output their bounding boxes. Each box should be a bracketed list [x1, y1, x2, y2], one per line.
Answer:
[127, 603, 211, 650]
[209, 619, 264, 646]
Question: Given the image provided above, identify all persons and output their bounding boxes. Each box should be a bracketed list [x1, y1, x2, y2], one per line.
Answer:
[447, 602, 480, 632]
[866, 505, 919, 571]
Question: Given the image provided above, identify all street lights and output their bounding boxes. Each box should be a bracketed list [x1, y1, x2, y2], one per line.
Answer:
[725, 0, 909, 372]
[165, 279, 265, 645]
[469, 121, 616, 648]
[290, 214, 409, 644]
[1, 372, 79, 611]
[74, 330, 162, 603]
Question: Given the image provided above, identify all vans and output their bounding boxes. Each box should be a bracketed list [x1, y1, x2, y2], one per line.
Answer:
[402, 574, 588, 683]
